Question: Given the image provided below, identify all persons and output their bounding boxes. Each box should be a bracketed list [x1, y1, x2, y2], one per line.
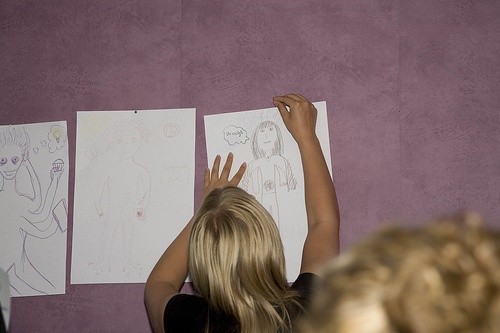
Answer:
[143, 92, 340, 333]
[292, 211, 499, 333]
